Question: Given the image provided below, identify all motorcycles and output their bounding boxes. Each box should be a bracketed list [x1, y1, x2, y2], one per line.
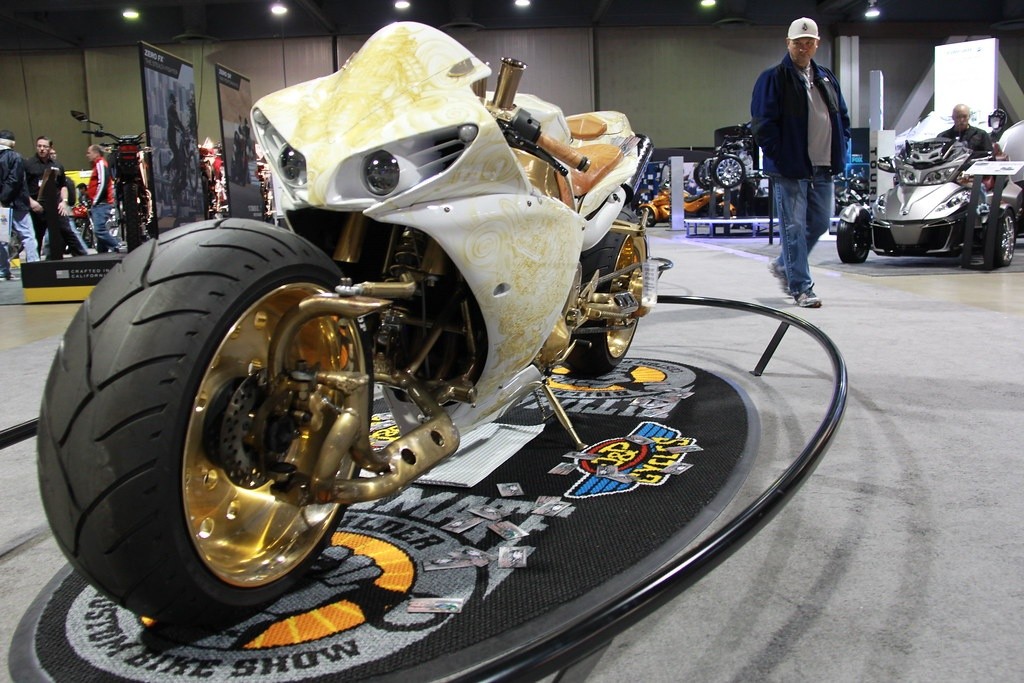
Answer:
[35, 21, 673, 630]
[70, 110, 148, 253]
[637, 179, 738, 229]
[693, 121, 769, 199]
[837, 121, 1024, 268]
[70, 200, 94, 249]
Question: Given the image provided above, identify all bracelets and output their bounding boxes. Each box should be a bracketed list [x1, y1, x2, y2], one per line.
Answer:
[63, 199, 68, 202]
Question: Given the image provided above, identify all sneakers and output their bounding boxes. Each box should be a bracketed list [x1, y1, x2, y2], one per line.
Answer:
[767, 258, 791, 295]
[794, 287, 822, 307]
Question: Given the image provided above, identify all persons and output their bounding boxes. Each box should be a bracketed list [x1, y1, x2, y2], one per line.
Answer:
[937, 104, 996, 178]
[0, 130, 94, 280]
[87, 144, 122, 253]
[163, 92, 198, 191]
[751, 17, 852, 307]
[234, 115, 253, 187]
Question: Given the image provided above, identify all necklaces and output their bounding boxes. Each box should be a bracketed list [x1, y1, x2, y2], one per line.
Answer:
[800, 64, 813, 89]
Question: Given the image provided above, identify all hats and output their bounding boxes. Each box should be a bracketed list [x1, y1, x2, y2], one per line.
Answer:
[76, 183, 87, 188]
[788, 17, 820, 40]
[0, 129, 15, 141]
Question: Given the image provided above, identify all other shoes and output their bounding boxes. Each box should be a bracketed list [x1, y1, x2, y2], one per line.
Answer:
[110, 243, 122, 251]
[0, 268, 10, 278]
